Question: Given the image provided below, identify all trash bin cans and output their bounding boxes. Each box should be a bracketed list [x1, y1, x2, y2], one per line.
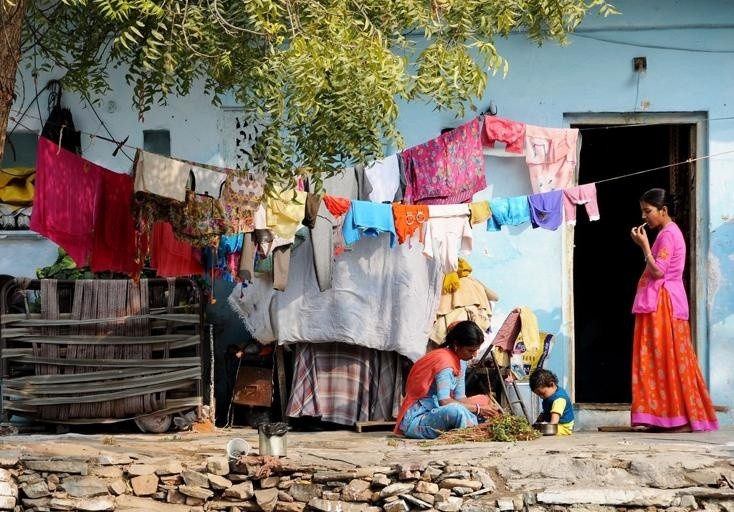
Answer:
[258, 422, 288, 457]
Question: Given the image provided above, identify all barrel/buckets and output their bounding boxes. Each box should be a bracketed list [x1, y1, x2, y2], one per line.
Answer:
[508, 382, 544, 426]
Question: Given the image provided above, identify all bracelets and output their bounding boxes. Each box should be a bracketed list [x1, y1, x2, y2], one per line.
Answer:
[475, 403, 480, 415]
[645, 252, 652, 262]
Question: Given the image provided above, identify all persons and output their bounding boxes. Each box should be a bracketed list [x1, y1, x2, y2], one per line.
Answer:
[529, 368, 574, 435]
[394, 320, 502, 440]
[630, 188, 720, 433]
